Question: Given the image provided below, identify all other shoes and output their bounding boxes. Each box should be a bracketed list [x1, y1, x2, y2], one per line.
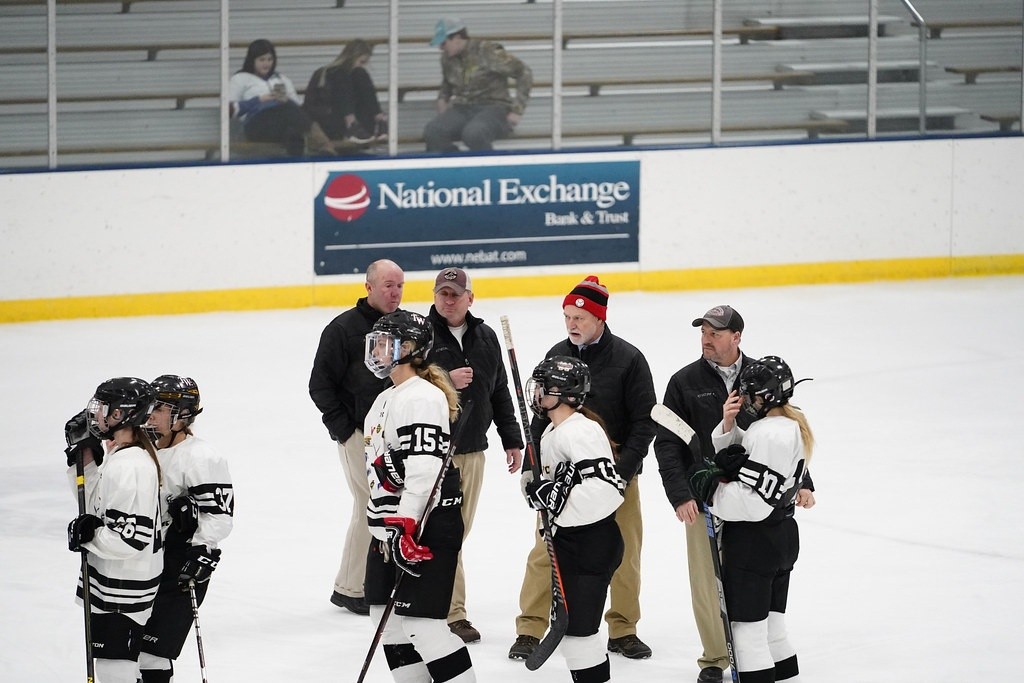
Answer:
[375, 118, 389, 144]
[341, 121, 376, 144]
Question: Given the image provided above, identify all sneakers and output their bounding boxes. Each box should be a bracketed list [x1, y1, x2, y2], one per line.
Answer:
[330, 590, 370, 616]
[697, 667, 723, 683]
[607, 635, 652, 659]
[448, 619, 480, 643]
[508, 635, 540, 659]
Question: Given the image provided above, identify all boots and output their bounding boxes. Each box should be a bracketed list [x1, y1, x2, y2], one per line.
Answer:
[301, 121, 338, 158]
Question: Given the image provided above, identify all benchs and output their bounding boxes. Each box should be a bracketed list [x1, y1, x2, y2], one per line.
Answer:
[0, 0, 1024, 167]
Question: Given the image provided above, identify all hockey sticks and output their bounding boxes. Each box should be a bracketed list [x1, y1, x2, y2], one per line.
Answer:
[75, 449, 98, 683]
[502, 313, 569, 673]
[189, 574, 211, 683]
[647, 402, 741, 683]
[357, 399, 478, 683]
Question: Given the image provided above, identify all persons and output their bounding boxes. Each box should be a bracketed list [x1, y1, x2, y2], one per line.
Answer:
[508, 276, 657, 660]
[139, 374, 235, 683]
[65, 377, 165, 683]
[686, 355, 814, 683]
[308, 258, 404, 617]
[421, 17, 534, 152]
[227, 39, 333, 158]
[361, 311, 478, 683]
[303, 38, 391, 143]
[525, 356, 626, 683]
[655, 303, 817, 683]
[422, 268, 526, 646]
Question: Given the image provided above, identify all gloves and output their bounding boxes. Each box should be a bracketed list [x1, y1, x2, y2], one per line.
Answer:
[688, 456, 721, 506]
[525, 479, 562, 515]
[714, 444, 749, 482]
[520, 470, 535, 504]
[384, 518, 433, 578]
[65, 408, 105, 468]
[177, 545, 221, 591]
[67, 514, 105, 553]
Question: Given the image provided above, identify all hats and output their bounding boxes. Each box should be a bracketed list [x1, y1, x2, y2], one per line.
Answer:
[692, 305, 744, 338]
[562, 276, 609, 321]
[429, 16, 466, 47]
[434, 267, 472, 295]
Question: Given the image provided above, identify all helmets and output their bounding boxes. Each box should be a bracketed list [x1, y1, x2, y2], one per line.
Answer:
[85, 376, 155, 440]
[373, 311, 434, 360]
[140, 374, 203, 441]
[740, 356, 794, 419]
[526, 355, 591, 419]
